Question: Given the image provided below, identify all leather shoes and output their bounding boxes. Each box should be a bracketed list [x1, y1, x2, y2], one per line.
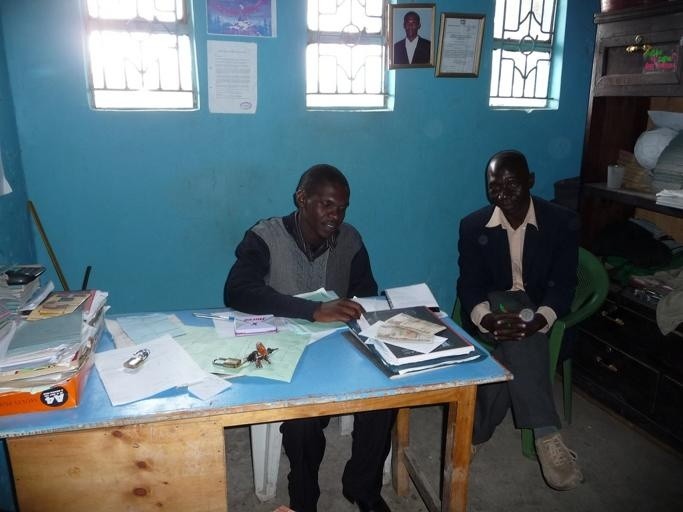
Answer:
[342, 486, 391, 512]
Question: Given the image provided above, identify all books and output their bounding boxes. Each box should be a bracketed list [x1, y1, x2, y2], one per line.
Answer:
[1, 266, 111, 395]
[341, 307, 488, 380]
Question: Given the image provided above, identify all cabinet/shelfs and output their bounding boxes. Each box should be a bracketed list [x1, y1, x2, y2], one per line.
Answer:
[552, 0, 683, 459]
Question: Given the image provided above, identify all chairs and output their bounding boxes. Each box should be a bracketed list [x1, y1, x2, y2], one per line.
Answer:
[451, 244, 610, 459]
[249, 415, 394, 501]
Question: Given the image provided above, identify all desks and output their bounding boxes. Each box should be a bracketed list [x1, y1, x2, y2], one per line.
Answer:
[0, 308, 515, 512]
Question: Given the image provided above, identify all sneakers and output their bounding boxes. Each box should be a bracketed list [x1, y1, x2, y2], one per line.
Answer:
[536, 433, 583, 491]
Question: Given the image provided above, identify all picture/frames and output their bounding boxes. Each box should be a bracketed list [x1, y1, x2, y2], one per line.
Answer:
[435, 12, 486, 78]
[385, 3, 436, 70]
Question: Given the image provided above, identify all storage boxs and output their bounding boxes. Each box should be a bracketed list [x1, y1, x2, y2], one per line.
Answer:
[1, 310, 105, 416]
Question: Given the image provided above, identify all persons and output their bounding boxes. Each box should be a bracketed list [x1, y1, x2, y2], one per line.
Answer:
[457, 151, 584, 491]
[394, 12, 430, 64]
[224, 165, 398, 512]
